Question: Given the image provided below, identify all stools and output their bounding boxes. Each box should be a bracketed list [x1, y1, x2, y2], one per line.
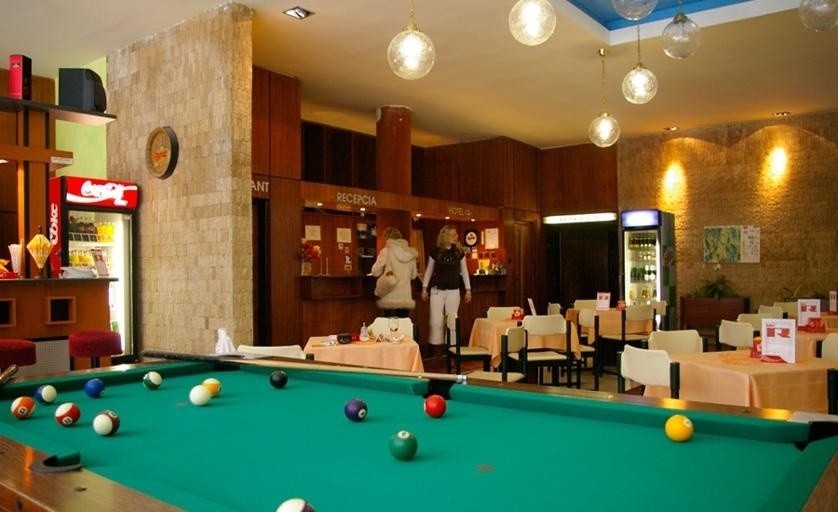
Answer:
[0, 340, 36, 368]
[69, 332, 122, 368]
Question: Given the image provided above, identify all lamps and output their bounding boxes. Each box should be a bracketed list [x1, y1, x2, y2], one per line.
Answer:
[661, 0, 703, 60]
[387, 0, 436, 80]
[621, 22, 658, 105]
[612, 0, 659, 22]
[508, 0, 556, 47]
[588, 48, 620, 148]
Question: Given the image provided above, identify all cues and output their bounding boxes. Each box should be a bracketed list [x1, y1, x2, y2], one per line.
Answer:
[140, 349, 469, 383]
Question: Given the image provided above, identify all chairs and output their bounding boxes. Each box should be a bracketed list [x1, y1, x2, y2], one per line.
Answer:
[236, 345, 305, 359]
[617, 344, 680, 398]
[508, 315, 571, 389]
[574, 300, 598, 309]
[487, 306, 524, 318]
[715, 319, 760, 350]
[548, 303, 561, 315]
[650, 300, 666, 316]
[755, 305, 788, 319]
[599, 305, 657, 378]
[559, 308, 599, 391]
[467, 326, 528, 383]
[447, 312, 492, 375]
[372, 317, 413, 337]
[816, 332, 838, 358]
[737, 314, 775, 331]
[642, 330, 709, 353]
[773, 302, 798, 317]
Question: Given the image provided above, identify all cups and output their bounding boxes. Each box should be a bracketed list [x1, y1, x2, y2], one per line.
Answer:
[388, 316, 400, 339]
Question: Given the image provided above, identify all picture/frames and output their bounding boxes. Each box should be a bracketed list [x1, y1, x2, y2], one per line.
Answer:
[704, 225, 760, 263]
[465, 230, 477, 247]
[145, 127, 179, 179]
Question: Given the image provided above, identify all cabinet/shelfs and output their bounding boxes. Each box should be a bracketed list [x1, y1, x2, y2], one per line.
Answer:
[0, 69, 119, 375]
[302, 182, 508, 358]
[680, 294, 750, 349]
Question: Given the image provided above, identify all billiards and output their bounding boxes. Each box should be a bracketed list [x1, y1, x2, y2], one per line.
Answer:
[83, 378, 105, 399]
[92, 409, 121, 437]
[55, 402, 81, 428]
[344, 398, 369, 423]
[142, 370, 163, 391]
[10, 395, 37, 419]
[34, 384, 58, 406]
[276, 497, 314, 512]
[388, 430, 419, 462]
[201, 377, 222, 398]
[189, 384, 212, 407]
[664, 413, 695, 443]
[269, 370, 288, 390]
[423, 393, 448, 419]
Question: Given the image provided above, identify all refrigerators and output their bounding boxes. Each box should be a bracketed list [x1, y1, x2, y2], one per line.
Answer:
[45, 175, 145, 364]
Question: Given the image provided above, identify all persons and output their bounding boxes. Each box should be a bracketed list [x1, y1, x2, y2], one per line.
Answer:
[421, 224, 471, 368]
[372, 226, 418, 319]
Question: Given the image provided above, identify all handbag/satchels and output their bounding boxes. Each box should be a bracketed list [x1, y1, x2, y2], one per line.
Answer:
[374, 253, 397, 296]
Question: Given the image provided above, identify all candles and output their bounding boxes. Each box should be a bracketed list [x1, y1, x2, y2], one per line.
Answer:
[320, 257, 322, 273]
[326, 256, 328, 273]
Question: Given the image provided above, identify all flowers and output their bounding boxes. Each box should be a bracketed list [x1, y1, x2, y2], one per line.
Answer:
[302, 261, 312, 276]
[302, 238, 322, 261]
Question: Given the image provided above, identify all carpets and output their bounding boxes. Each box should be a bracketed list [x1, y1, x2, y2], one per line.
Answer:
[625, 385, 644, 395]
[452, 359, 483, 372]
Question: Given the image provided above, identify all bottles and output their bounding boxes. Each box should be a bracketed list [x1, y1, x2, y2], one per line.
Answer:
[631, 251, 657, 265]
[641, 286, 647, 302]
[70, 249, 94, 268]
[653, 289, 657, 301]
[69, 216, 116, 241]
[628, 233, 658, 251]
[632, 267, 657, 282]
